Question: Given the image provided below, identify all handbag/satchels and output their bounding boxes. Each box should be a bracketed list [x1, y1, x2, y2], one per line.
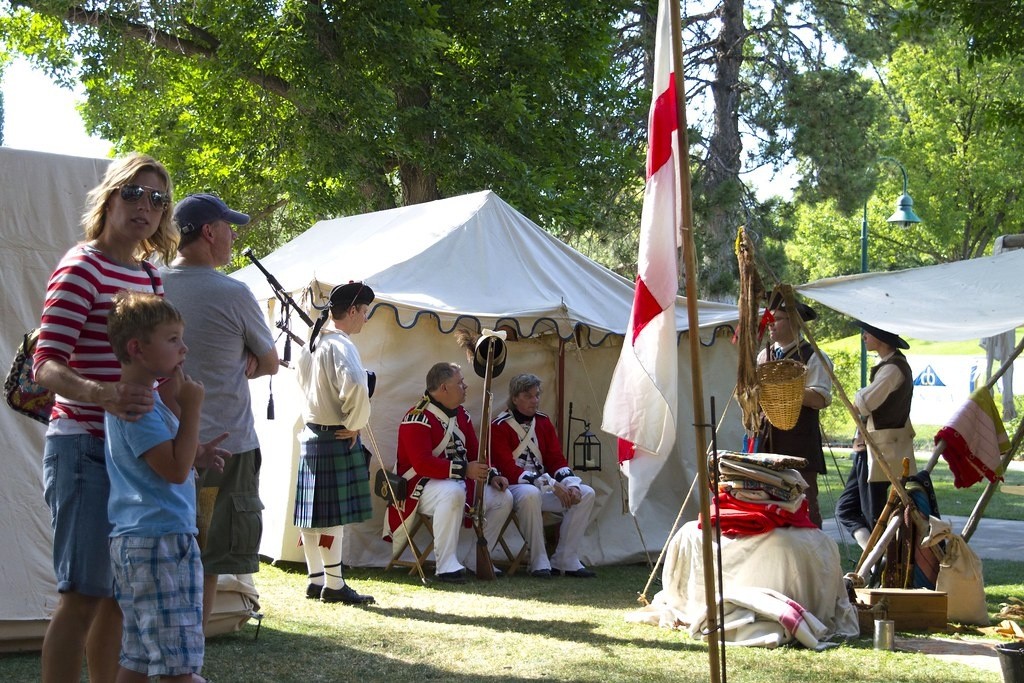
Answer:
[374, 469, 407, 501]
[866, 428, 918, 483]
[4, 328, 56, 425]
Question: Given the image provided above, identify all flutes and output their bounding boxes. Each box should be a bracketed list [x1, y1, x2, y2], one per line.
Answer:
[240, 244, 377, 400]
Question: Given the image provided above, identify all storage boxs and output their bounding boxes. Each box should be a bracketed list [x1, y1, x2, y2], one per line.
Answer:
[855, 588, 948, 631]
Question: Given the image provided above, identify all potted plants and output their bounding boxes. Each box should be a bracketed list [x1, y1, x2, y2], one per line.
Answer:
[870, 595, 894, 651]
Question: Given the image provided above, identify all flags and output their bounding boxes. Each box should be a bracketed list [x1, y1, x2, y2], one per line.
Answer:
[600, 1, 683, 517]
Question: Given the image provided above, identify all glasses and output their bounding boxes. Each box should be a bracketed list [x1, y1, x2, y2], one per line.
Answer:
[116, 183, 172, 211]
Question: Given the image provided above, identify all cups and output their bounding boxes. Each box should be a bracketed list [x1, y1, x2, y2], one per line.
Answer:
[875, 619, 895, 650]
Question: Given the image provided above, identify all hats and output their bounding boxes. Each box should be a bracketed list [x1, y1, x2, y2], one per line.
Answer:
[172, 193, 249, 233]
[766, 290, 817, 322]
[456, 328, 507, 378]
[310, 283, 374, 352]
[851, 319, 910, 349]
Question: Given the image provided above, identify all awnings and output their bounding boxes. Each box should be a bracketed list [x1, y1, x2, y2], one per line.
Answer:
[792, 247, 1024, 343]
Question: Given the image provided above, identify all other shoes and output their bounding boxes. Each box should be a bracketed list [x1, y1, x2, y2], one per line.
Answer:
[532, 569, 552, 579]
[869, 556, 886, 589]
[438, 571, 469, 584]
[551, 568, 597, 578]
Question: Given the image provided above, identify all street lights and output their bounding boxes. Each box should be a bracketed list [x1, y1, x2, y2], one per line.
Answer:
[860, 156, 922, 422]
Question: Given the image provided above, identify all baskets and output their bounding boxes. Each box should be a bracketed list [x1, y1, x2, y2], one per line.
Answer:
[754, 358, 808, 431]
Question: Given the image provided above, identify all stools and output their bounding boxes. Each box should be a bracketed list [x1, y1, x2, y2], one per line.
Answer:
[384, 507, 551, 577]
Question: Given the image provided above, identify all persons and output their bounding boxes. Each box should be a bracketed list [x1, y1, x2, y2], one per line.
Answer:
[293, 281, 375, 604]
[835, 319, 918, 589]
[485, 374, 598, 579]
[382, 361, 513, 583]
[155, 196, 278, 635]
[757, 290, 834, 529]
[33, 154, 212, 683]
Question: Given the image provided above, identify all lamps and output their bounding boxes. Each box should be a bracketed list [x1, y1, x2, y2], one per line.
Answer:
[566, 402, 602, 472]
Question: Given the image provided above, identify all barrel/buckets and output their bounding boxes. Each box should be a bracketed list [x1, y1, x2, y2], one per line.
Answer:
[995, 640, 1024, 683]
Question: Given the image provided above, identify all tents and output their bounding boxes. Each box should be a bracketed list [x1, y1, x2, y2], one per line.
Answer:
[226, 189, 767, 568]
[0, 148, 114, 621]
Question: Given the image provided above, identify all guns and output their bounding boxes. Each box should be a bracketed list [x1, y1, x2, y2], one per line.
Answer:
[466, 335, 500, 582]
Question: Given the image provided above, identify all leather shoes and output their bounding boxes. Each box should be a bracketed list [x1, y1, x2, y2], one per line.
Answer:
[320, 585, 375, 604]
[306, 583, 325, 598]
